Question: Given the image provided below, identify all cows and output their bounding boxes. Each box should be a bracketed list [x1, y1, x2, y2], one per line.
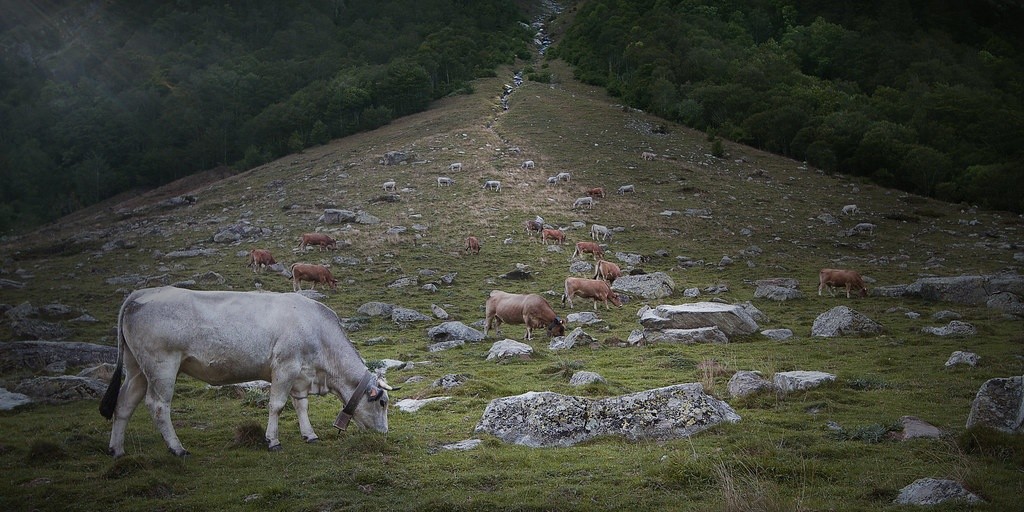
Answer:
[483, 289, 565, 341]
[247, 249, 278, 270]
[570, 241, 607, 262]
[287, 263, 338, 293]
[584, 188, 606, 198]
[572, 196, 593, 210]
[590, 223, 613, 241]
[617, 185, 636, 196]
[591, 260, 621, 284]
[381, 181, 396, 192]
[816, 268, 869, 299]
[541, 230, 566, 246]
[525, 220, 544, 235]
[437, 160, 570, 192]
[464, 236, 481, 256]
[98, 286, 401, 458]
[298, 232, 337, 252]
[561, 278, 623, 312]
[640, 151, 657, 161]
